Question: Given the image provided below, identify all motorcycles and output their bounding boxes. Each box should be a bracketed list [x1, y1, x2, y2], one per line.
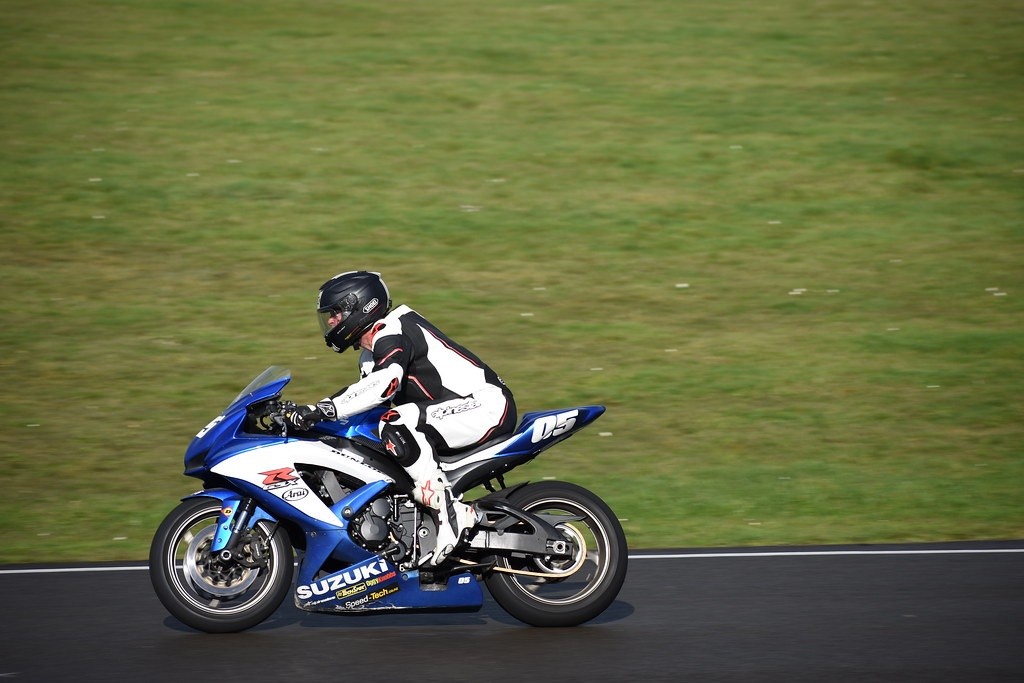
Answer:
[147, 363, 629, 636]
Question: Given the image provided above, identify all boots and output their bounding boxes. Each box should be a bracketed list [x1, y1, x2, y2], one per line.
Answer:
[412, 472, 478, 565]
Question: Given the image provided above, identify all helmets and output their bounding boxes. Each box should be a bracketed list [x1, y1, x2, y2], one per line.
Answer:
[317, 270, 393, 353]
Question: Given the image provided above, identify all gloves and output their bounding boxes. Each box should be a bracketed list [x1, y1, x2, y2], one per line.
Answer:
[289, 397, 337, 427]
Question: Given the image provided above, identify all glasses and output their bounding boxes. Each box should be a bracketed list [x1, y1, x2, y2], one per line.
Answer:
[330, 310, 341, 316]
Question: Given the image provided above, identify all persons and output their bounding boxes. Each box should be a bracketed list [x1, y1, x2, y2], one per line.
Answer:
[281, 271, 517, 566]
